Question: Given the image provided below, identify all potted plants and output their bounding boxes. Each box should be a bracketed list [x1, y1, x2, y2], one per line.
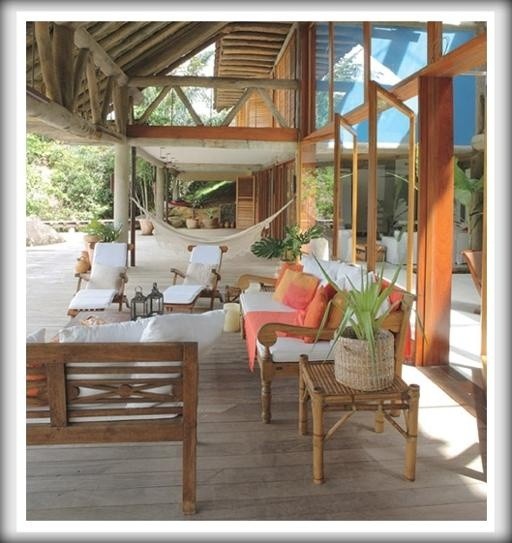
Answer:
[305, 248, 432, 392]
[86, 216, 124, 268]
[247, 222, 325, 275]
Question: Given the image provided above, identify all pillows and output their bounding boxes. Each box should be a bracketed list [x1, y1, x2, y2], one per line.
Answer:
[270, 252, 393, 345]
[86, 262, 128, 291]
[56, 315, 156, 400]
[26, 324, 47, 347]
[127, 303, 230, 413]
[183, 260, 218, 287]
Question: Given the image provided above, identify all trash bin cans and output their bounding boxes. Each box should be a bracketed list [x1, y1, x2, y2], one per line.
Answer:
[223, 303, 240, 332]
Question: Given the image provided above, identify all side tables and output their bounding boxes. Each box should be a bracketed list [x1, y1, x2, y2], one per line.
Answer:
[296, 353, 421, 486]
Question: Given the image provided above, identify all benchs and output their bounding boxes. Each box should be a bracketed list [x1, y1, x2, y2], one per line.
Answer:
[235, 252, 415, 426]
[26, 340, 203, 515]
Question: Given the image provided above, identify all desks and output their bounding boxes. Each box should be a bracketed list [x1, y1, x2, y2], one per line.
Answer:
[355, 243, 386, 262]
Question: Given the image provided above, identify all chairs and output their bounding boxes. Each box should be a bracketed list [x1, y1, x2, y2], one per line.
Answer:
[338, 229, 355, 264]
[383, 227, 419, 264]
[66, 241, 132, 320]
[461, 248, 484, 313]
[161, 241, 229, 318]
